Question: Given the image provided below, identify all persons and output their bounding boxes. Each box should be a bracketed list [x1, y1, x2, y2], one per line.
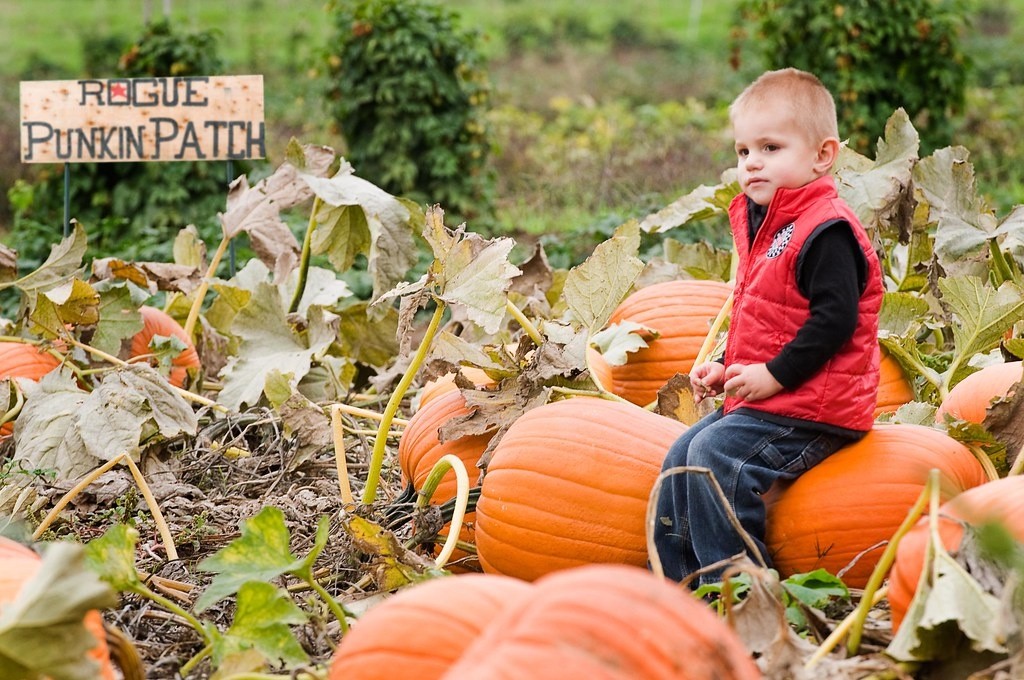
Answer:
[649, 66, 884, 599]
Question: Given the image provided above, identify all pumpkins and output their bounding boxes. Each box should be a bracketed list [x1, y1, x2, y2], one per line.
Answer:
[326, 281, 1024, 680]
[0, 536, 112, 680]
[0, 305, 204, 444]
